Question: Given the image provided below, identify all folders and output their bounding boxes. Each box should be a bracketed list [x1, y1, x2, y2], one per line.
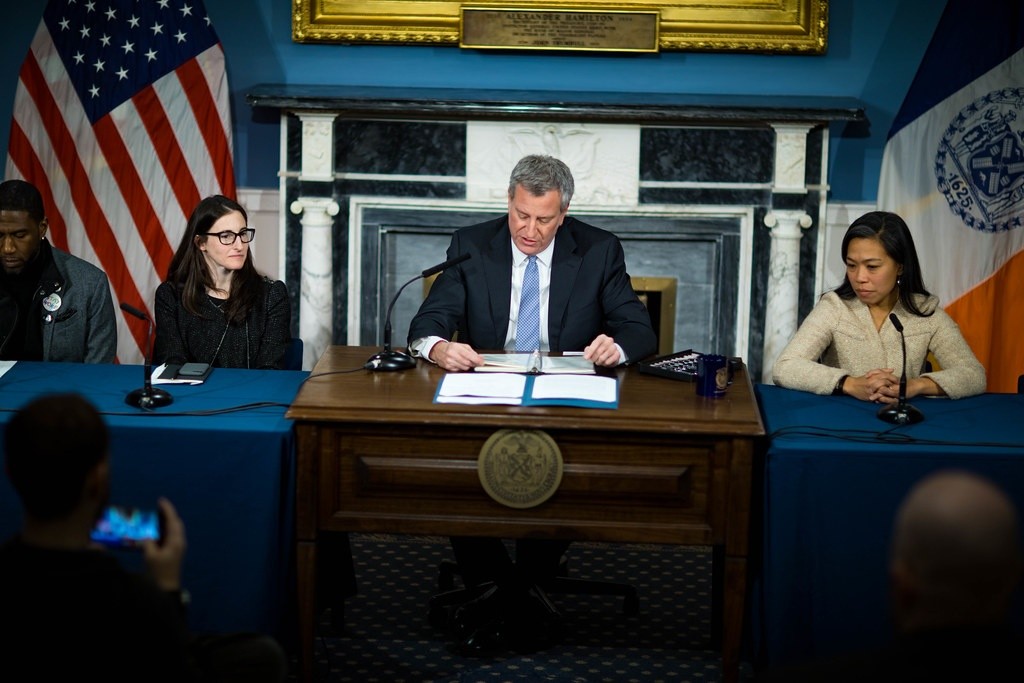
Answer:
[433, 372, 619, 410]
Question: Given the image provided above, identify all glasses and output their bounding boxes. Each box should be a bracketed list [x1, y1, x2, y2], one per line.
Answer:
[197, 228, 256, 245]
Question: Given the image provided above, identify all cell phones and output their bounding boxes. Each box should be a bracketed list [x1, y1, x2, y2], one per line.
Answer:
[179, 363, 209, 376]
[89, 502, 168, 549]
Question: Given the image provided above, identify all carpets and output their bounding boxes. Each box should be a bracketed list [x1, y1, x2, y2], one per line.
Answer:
[286, 533, 756, 683]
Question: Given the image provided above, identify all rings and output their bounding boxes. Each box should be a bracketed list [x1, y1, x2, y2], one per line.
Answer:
[889, 384, 894, 390]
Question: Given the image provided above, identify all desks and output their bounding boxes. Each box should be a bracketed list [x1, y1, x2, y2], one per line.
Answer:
[743, 382, 1024, 671]
[0, 361, 358, 637]
[286, 343, 766, 682]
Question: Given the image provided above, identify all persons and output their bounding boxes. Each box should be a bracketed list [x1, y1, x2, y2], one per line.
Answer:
[0, 395, 188, 683]
[771, 211, 987, 404]
[153, 195, 292, 370]
[745, 469, 1024, 683]
[407, 156, 657, 660]
[0, 180, 117, 364]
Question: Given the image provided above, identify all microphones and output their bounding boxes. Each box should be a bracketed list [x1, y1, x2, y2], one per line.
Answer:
[119, 302, 173, 408]
[365, 251, 472, 371]
[876, 314, 924, 424]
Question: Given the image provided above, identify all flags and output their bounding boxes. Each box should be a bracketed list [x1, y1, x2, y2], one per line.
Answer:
[877, 0, 1024, 394]
[3, 0, 237, 366]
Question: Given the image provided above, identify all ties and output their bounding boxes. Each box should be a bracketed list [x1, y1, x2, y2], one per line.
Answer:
[516, 254, 540, 352]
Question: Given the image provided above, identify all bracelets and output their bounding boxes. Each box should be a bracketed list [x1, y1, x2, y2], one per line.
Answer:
[838, 374, 851, 395]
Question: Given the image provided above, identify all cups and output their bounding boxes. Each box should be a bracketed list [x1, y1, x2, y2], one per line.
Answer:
[697, 354, 737, 398]
